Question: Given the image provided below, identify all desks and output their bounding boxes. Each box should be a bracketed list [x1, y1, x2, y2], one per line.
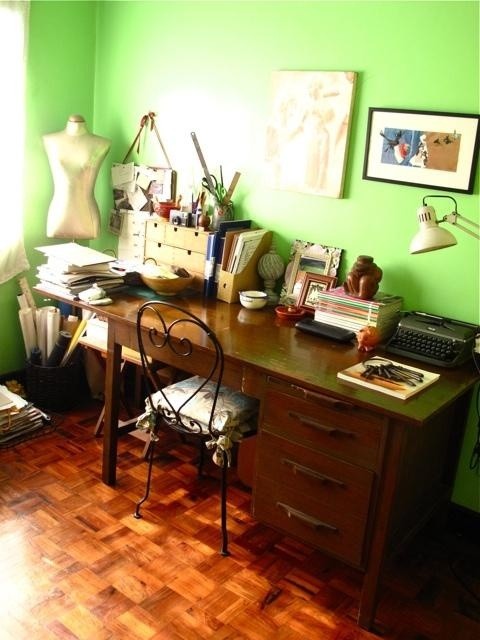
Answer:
[31, 283, 480, 630]
[80, 336, 171, 461]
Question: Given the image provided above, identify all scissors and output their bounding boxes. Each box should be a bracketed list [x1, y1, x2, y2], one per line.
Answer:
[202, 174, 227, 205]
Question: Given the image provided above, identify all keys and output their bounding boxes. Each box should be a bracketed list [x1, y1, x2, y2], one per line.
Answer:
[360, 357, 424, 387]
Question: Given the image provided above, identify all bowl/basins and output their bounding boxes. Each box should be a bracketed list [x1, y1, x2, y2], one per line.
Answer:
[153, 201, 181, 222]
[238, 290, 270, 310]
[139, 272, 196, 297]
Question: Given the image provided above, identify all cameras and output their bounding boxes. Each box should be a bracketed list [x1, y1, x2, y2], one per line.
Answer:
[169, 209, 192, 227]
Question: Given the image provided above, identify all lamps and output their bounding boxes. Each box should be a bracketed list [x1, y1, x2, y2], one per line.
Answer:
[408, 195, 462, 255]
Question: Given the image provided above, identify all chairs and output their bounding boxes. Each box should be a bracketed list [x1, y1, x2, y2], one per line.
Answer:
[132, 299, 258, 555]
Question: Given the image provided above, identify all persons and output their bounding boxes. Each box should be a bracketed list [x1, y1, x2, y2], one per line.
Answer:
[41, 115, 114, 239]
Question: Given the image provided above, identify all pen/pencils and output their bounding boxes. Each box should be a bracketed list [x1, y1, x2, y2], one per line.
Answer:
[112, 267, 125, 271]
[192, 191, 207, 207]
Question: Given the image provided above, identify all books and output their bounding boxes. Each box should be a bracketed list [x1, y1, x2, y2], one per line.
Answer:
[0, 382, 48, 447]
[33, 243, 131, 301]
[203, 218, 268, 299]
[313, 286, 404, 337]
[337, 353, 442, 401]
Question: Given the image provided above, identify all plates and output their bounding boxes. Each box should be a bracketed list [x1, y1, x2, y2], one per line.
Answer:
[274, 305, 306, 320]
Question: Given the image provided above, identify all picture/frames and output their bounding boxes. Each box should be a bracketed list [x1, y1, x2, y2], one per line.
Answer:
[279, 239, 343, 305]
[362, 106, 480, 195]
[298, 272, 341, 315]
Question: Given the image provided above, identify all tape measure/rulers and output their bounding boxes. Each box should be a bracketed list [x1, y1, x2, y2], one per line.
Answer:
[191, 131, 214, 195]
[222, 171, 240, 206]
